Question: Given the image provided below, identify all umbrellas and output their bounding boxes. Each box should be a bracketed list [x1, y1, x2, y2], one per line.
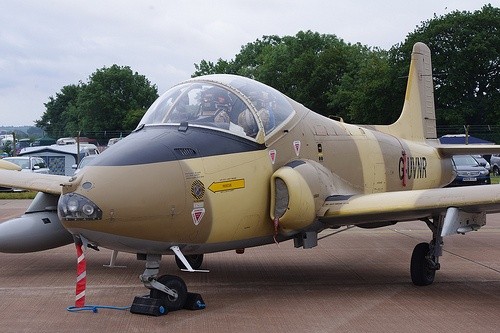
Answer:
[439, 134, 489, 144]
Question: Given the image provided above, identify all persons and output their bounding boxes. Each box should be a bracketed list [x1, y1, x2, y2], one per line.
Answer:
[261, 89, 276, 111]
[239, 92, 270, 138]
[196, 91, 230, 125]
[217, 90, 232, 112]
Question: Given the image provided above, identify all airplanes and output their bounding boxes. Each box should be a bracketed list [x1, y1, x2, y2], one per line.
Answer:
[0, 42, 500, 317]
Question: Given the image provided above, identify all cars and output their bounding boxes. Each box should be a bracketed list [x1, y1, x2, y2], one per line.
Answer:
[445, 154, 491, 187]
[472, 154, 491, 173]
[76, 144, 100, 167]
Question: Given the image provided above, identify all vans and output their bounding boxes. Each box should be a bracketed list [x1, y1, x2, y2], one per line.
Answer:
[56, 137, 77, 145]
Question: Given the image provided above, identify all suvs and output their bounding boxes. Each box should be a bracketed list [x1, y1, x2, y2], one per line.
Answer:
[489, 151, 500, 177]
